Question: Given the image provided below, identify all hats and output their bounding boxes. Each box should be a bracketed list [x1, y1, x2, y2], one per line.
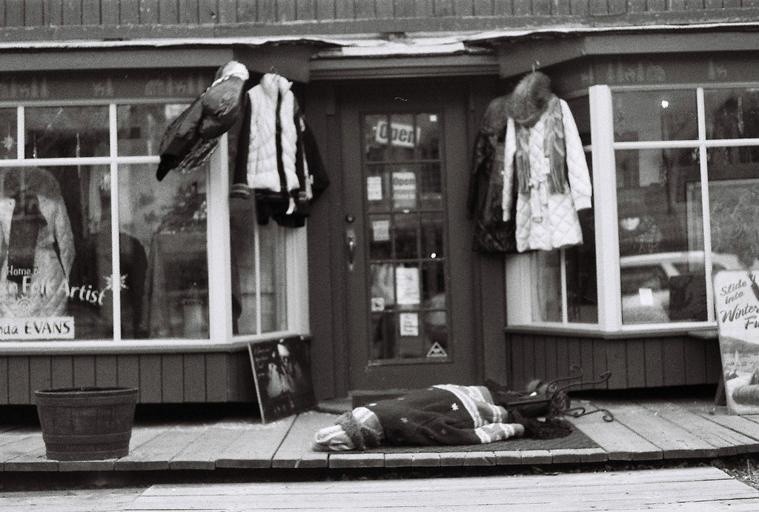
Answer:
[504, 72, 551, 127]
[480, 94, 508, 134]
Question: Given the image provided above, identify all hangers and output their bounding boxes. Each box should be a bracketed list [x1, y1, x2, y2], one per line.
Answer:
[270, 64, 281, 86]
[530, 58, 540, 73]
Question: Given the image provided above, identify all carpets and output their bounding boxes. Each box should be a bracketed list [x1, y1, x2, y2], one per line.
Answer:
[324, 394, 602, 454]
